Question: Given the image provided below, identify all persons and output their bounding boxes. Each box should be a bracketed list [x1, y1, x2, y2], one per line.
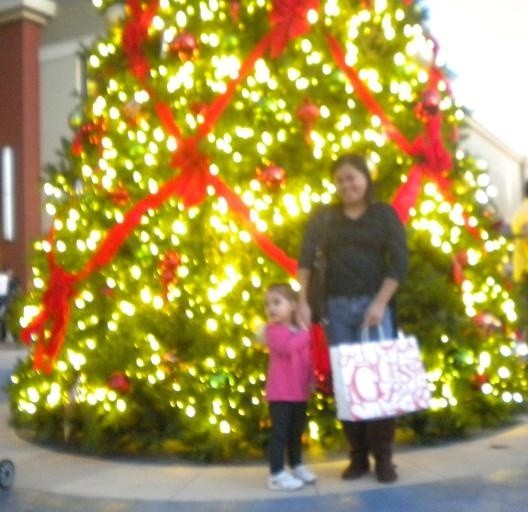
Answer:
[295, 153, 408, 483]
[260, 281, 321, 493]
[0, 266, 22, 342]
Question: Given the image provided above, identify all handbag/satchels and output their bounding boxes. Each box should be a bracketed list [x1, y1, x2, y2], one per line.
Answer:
[306, 203, 330, 325]
[328, 319, 431, 425]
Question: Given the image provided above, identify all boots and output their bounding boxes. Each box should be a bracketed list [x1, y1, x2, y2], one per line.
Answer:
[339, 449, 371, 482]
[373, 449, 400, 484]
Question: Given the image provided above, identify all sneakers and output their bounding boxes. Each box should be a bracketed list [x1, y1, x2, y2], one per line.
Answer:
[266, 468, 305, 493]
[288, 462, 319, 485]
